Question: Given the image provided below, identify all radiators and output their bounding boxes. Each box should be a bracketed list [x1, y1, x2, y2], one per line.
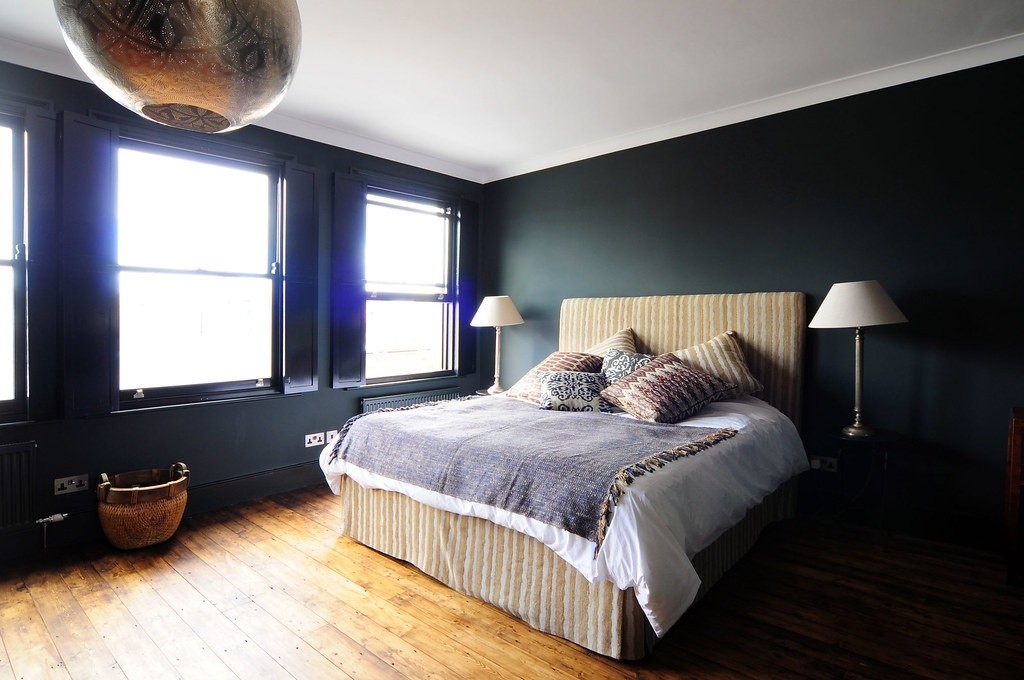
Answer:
[360, 387, 460, 413]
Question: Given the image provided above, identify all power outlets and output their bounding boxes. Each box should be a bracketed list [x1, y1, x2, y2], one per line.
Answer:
[326, 429, 338, 443]
[304, 432, 324, 448]
[54, 474, 88, 495]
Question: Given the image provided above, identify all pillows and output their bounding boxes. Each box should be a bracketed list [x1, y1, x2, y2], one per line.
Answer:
[585, 328, 636, 357]
[599, 352, 738, 425]
[504, 352, 604, 405]
[600, 347, 658, 407]
[659, 330, 765, 396]
[535, 370, 614, 414]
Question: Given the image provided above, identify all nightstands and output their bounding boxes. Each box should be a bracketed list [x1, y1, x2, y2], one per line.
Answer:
[804, 428, 906, 552]
[475, 389, 509, 395]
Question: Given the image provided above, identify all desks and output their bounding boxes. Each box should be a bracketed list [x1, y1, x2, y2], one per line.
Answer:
[1004, 405, 1024, 586]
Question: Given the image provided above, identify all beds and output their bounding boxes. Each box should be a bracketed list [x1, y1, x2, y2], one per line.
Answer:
[319, 291, 806, 661]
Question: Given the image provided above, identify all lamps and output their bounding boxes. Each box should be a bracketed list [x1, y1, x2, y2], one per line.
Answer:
[808, 280, 909, 438]
[52, 0, 303, 134]
[469, 296, 526, 395]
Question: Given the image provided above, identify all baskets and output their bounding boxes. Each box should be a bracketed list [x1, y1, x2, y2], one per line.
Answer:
[98, 462, 190, 551]
